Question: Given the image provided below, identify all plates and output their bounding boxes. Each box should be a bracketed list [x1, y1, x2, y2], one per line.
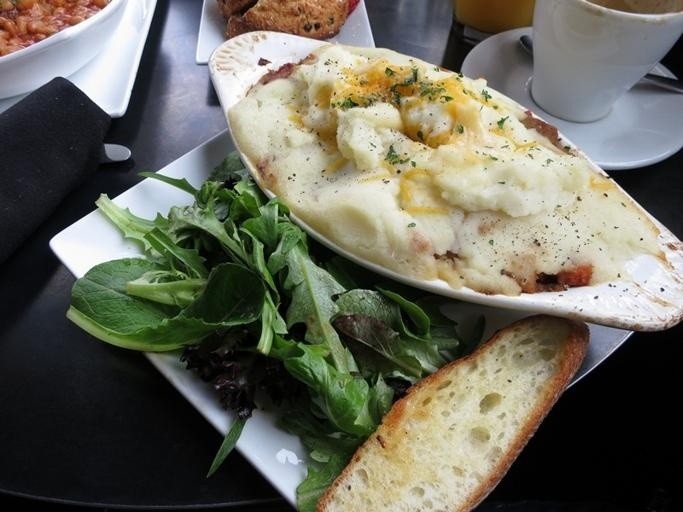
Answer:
[47, 28, 683, 512]
[195, 0, 377, 67]
[459, 24, 683, 170]
[1, 0, 157, 119]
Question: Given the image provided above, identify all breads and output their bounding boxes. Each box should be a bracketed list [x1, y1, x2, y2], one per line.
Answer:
[315, 311, 591, 512]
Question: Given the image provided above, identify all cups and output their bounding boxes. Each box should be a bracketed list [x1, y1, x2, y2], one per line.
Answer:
[530, 0, 682, 124]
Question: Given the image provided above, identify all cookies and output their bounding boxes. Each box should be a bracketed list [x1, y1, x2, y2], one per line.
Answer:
[215, 0, 251, 19]
[226, 1, 362, 40]
